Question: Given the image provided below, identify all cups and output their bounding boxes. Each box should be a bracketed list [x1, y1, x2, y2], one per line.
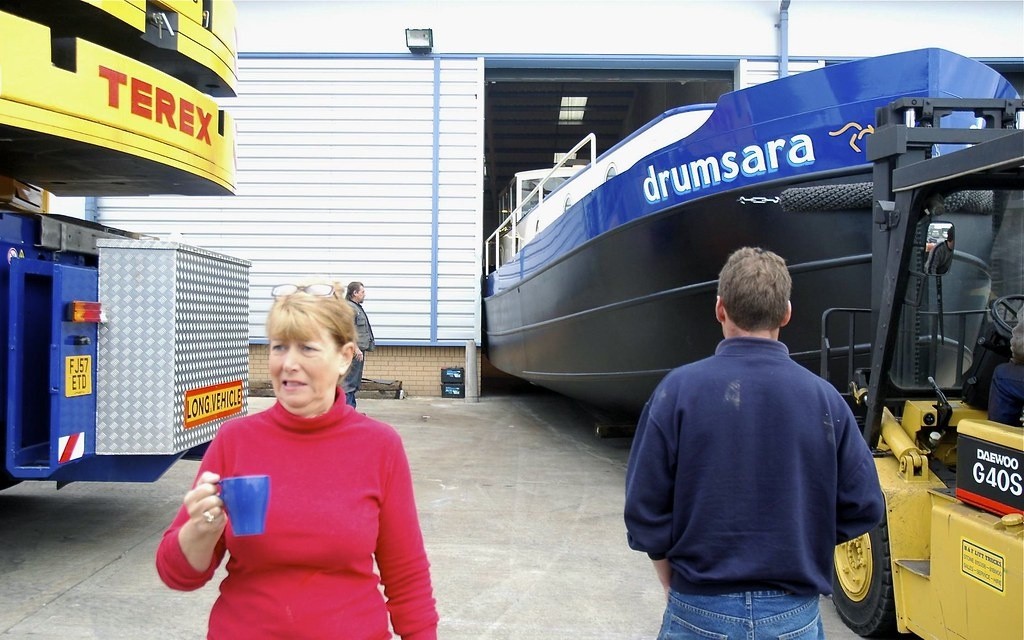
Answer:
[211, 474, 270, 537]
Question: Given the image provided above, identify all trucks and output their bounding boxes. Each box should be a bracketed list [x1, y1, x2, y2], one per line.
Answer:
[1, 0, 252, 484]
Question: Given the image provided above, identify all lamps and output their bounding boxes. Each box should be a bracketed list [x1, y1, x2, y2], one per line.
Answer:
[406, 27, 435, 54]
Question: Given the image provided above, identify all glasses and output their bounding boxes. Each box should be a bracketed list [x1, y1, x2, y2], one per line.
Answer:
[271, 284, 338, 299]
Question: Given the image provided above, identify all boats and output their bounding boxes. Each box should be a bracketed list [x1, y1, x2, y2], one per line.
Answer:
[481, 47, 1023, 415]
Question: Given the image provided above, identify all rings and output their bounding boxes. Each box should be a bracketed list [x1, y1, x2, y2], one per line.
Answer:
[204, 511, 214, 523]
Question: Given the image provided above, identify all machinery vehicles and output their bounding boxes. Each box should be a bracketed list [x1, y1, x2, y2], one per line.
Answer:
[822, 98, 1024, 640]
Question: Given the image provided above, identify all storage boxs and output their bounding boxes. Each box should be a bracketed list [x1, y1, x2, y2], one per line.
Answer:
[441, 367, 464, 383]
[441, 384, 465, 398]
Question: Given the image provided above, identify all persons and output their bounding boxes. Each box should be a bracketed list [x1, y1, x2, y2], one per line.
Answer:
[156, 280, 439, 640]
[948, 304, 1024, 473]
[623, 247, 885, 640]
[337, 282, 375, 416]
[925, 227, 955, 276]
